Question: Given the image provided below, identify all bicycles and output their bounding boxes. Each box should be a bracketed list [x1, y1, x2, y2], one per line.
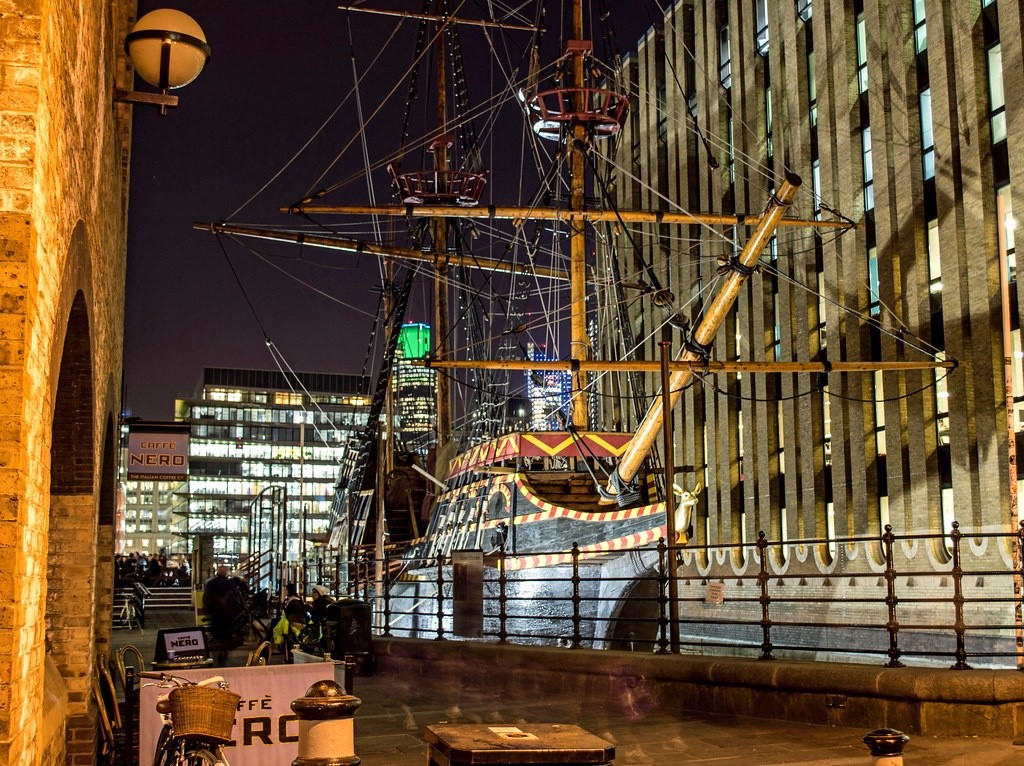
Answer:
[120, 593, 138, 630]
[137, 672, 241, 766]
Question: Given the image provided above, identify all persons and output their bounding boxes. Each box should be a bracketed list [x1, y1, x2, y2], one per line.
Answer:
[202, 566, 237, 667]
[115, 551, 189, 588]
[259, 583, 336, 655]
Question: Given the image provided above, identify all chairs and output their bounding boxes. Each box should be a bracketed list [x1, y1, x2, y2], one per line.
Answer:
[91, 645, 145, 766]
[246, 641, 273, 666]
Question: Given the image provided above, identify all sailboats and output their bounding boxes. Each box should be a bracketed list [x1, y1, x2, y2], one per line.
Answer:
[193, 0, 961, 654]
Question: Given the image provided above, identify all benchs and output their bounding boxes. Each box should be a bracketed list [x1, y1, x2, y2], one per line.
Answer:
[149, 576, 158, 580]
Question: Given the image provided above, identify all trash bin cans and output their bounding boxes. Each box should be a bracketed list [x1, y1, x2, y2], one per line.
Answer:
[424, 723, 615, 766]
[326, 599, 372, 661]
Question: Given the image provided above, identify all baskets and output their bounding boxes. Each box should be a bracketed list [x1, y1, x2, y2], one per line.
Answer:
[169, 687, 241, 743]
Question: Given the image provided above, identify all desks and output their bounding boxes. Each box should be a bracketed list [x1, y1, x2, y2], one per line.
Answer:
[153, 567, 183, 587]
[151, 658, 213, 671]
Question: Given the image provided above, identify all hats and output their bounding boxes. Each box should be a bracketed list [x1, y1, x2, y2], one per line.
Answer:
[314, 585, 329, 596]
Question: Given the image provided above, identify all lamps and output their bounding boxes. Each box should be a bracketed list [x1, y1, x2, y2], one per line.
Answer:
[113, 9, 211, 117]
